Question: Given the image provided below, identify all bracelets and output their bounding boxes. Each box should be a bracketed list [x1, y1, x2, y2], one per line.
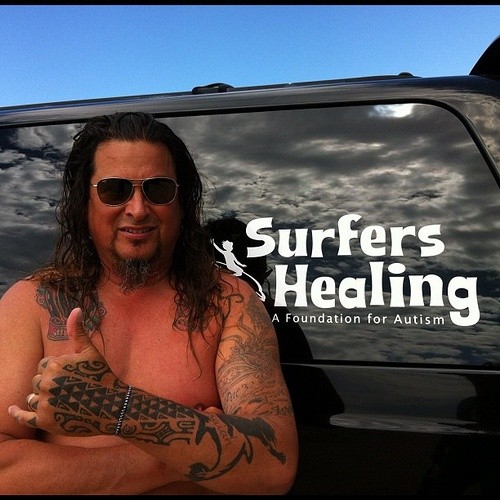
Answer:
[114, 384, 132, 439]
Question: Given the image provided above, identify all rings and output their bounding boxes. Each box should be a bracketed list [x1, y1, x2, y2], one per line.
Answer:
[26, 393, 39, 412]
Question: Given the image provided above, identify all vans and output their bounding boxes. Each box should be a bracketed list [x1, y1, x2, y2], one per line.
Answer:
[1, 72, 498, 498]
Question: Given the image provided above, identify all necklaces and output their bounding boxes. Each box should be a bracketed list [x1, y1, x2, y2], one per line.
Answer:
[0, 108, 301, 500]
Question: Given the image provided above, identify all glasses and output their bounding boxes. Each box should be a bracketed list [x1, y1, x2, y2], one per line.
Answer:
[88, 176, 179, 206]
